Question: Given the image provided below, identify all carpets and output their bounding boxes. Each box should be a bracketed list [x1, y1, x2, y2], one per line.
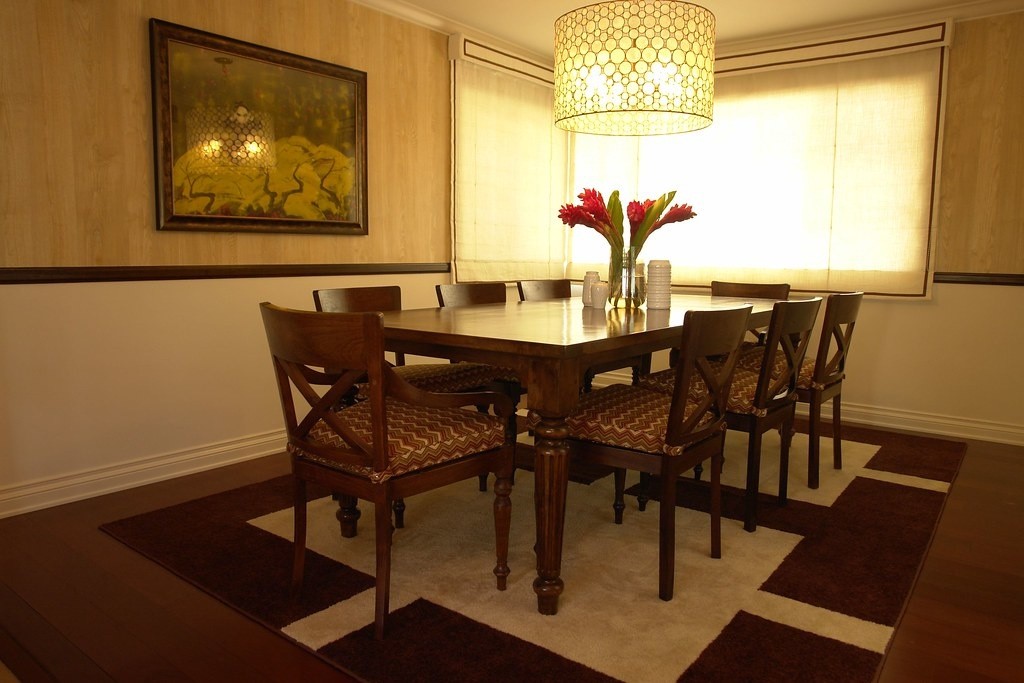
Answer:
[98, 407, 969, 683]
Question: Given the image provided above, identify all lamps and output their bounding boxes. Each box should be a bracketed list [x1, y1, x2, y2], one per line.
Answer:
[554, 0, 717, 137]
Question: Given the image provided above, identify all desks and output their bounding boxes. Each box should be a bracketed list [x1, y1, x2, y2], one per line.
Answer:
[382, 293, 782, 615]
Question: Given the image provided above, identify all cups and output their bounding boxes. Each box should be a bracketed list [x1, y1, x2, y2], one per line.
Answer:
[646, 259, 672, 311]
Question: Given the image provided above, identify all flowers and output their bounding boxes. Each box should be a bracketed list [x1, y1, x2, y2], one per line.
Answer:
[558, 188, 698, 310]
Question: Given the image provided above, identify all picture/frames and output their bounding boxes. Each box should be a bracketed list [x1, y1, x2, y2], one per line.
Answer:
[150, 17, 369, 235]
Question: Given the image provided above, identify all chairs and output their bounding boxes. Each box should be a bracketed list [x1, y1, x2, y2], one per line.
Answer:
[259, 283, 528, 641]
[518, 280, 864, 601]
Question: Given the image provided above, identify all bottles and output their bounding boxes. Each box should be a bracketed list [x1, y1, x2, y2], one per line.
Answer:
[591, 282, 609, 309]
[582, 271, 601, 307]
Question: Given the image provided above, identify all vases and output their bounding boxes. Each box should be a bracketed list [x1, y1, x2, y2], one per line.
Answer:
[607, 247, 649, 309]
